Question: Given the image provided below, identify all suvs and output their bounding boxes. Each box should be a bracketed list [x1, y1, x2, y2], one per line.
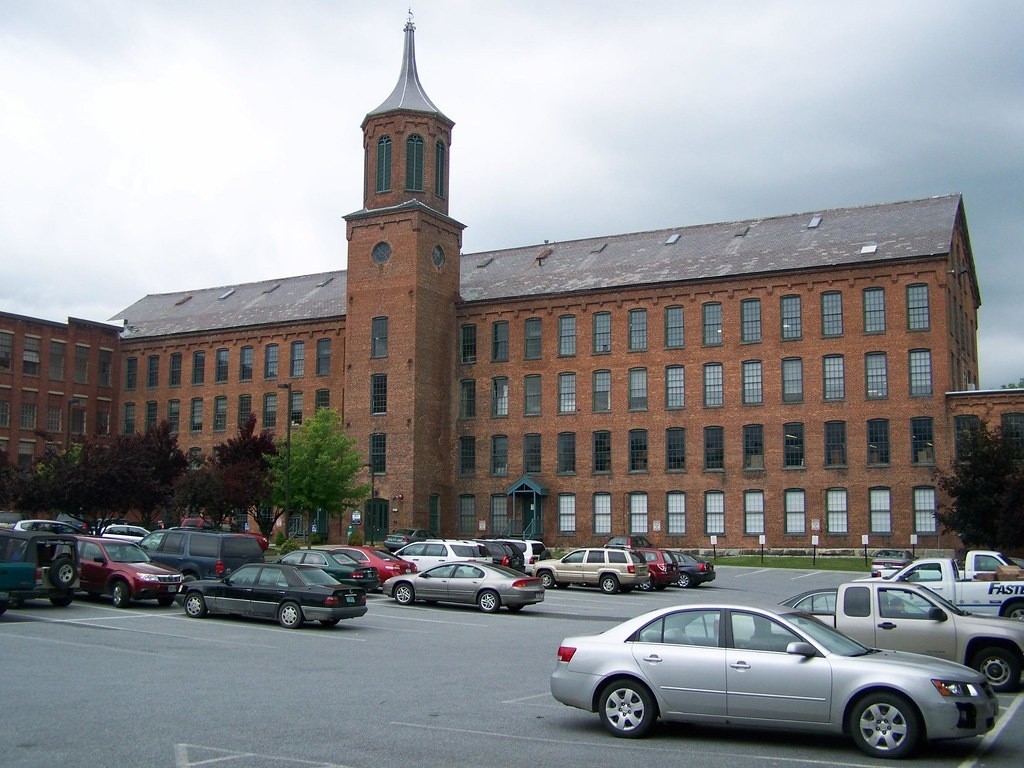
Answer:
[472, 540, 526, 575]
[180, 516, 269, 553]
[0, 529, 82, 606]
[391, 539, 492, 575]
[532, 546, 650, 595]
[503, 538, 546, 573]
[604, 535, 652, 551]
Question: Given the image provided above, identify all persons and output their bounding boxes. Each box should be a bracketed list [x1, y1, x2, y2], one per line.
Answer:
[753, 622, 773, 639]
[886, 598, 911, 618]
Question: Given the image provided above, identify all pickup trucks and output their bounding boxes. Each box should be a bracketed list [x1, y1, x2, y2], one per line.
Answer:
[877, 550, 1022, 581]
[852, 557, 1024, 619]
[714, 581, 1024, 690]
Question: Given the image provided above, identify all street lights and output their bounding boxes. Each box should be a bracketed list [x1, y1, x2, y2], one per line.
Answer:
[362, 462, 375, 547]
[276, 381, 292, 541]
[65, 398, 83, 459]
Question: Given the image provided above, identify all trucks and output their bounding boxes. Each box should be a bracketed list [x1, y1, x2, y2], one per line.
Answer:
[0, 563, 43, 617]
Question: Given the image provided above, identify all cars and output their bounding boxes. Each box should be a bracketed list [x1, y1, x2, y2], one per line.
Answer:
[872, 547, 915, 578]
[381, 561, 546, 613]
[311, 545, 418, 585]
[271, 550, 382, 593]
[637, 547, 682, 591]
[173, 564, 368, 629]
[550, 601, 1000, 758]
[14, 519, 154, 543]
[68, 535, 184, 609]
[779, 589, 928, 617]
[383, 528, 435, 551]
[668, 551, 716, 588]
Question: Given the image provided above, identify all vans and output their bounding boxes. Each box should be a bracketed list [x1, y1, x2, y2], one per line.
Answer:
[119, 530, 267, 592]
